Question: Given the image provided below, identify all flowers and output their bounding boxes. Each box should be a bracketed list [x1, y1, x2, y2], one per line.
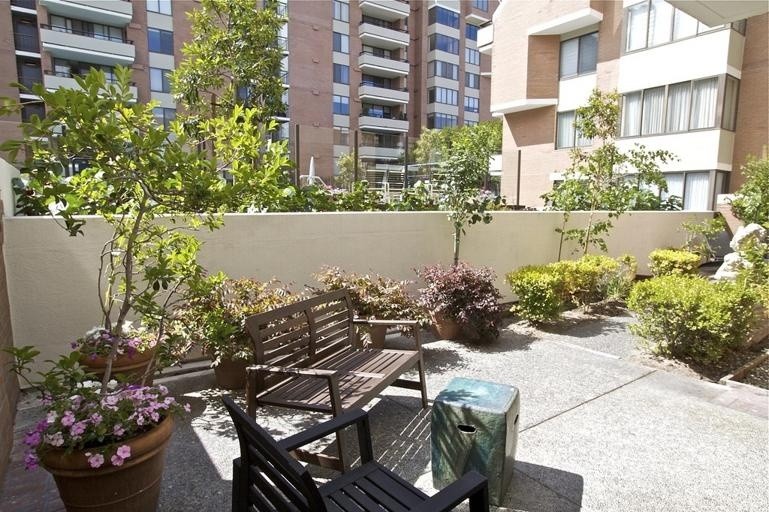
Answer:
[1, 318, 193, 472]
[170, 275, 308, 369]
[412, 255, 504, 344]
[316, 264, 432, 330]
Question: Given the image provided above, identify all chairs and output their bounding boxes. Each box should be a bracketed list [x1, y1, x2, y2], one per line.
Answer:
[222, 398, 492, 511]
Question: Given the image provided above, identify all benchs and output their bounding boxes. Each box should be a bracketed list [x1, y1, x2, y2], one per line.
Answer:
[240, 287, 428, 476]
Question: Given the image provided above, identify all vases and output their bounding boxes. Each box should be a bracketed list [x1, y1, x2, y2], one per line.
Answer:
[38, 413, 177, 512]
[210, 355, 249, 389]
[355, 322, 388, 349]
[429, 311, 463, 341]
